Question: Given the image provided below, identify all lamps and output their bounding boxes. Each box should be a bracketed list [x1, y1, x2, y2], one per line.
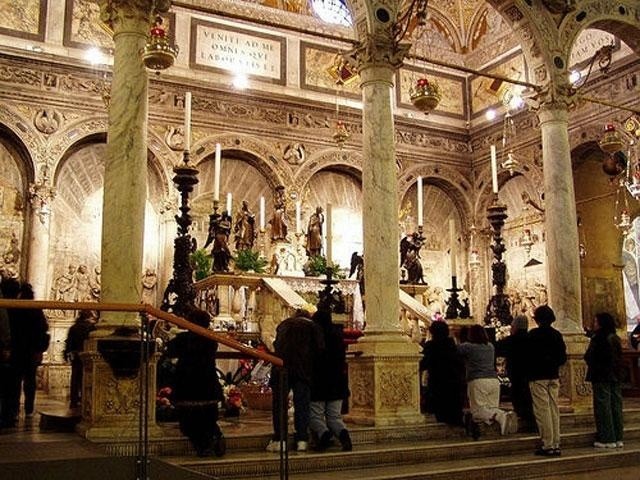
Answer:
[597, 124, 625, 158]
[326, 53, 357, 85]
[140, 0, 178, 69]
[409, 0, 442, 115]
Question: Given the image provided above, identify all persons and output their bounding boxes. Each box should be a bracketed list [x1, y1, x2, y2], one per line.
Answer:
[272, 190, 288, 241]
[212, 211, 232, 271]
[584, 312, 625, 449]
[266, 311, 318, 451]
[307, 307, 355, 452]
[162, 310, 226, 457]
[63, 310, 96, 408]
[420, 319, 518, 438]
[0, 278, 50, 429]
[525, 305, 567, 455]
[495, 316, 539, 434]
[400, 231, 425, 284]
[307, 206, 324, 259]
[234, 200, 256, 251]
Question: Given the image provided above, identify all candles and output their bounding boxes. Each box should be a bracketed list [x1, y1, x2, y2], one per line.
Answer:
[213, 142, 221, 202]
[227, 193, 232, 216]
[295, 200, 302, 233]
[415, 175, 424, 226]
[258, 197, 266, 229]
[184, 91, 193, 152]
[490, 144, 499, 194]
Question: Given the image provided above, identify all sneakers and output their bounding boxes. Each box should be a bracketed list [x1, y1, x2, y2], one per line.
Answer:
[535, 445, 561, 456]
[297, 441, 308, 451]
[500, 411, 518, 436]
[266, 440, 286, 452]
[593, 441, 624, 448]
